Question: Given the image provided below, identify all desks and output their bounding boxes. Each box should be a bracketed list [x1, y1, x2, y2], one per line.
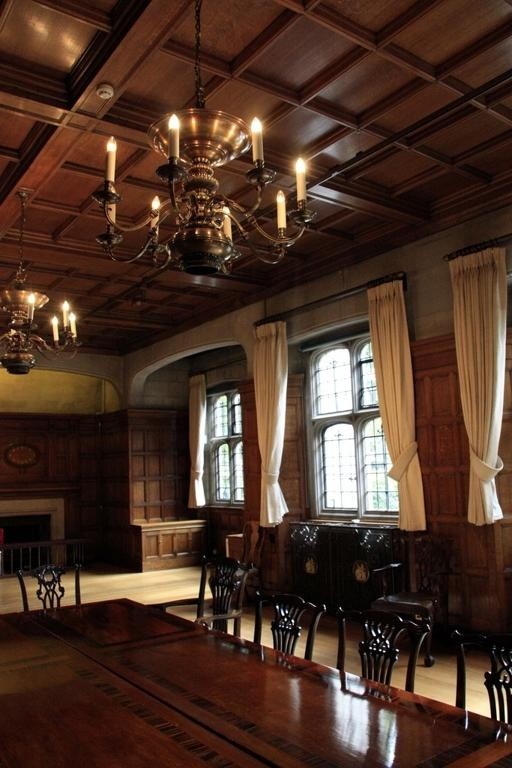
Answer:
[0, 598, 512, 767]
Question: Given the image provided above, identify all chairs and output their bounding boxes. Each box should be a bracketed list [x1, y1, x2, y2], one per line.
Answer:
[254, 591, 326, 661]
[452, 629, 512, 725]
[371, 528, 454, 666]
[146, 555, 253, 637]
[207, 522, 264, 614]
[18, 563, 82, 612]
[337, 606, 430, 692]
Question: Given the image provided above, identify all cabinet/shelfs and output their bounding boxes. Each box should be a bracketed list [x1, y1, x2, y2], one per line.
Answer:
[289, 521, 400, 626]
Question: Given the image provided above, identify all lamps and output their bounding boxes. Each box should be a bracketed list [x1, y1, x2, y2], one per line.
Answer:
[0, 187, 83, 375]
[91, 0, 317, 279]
